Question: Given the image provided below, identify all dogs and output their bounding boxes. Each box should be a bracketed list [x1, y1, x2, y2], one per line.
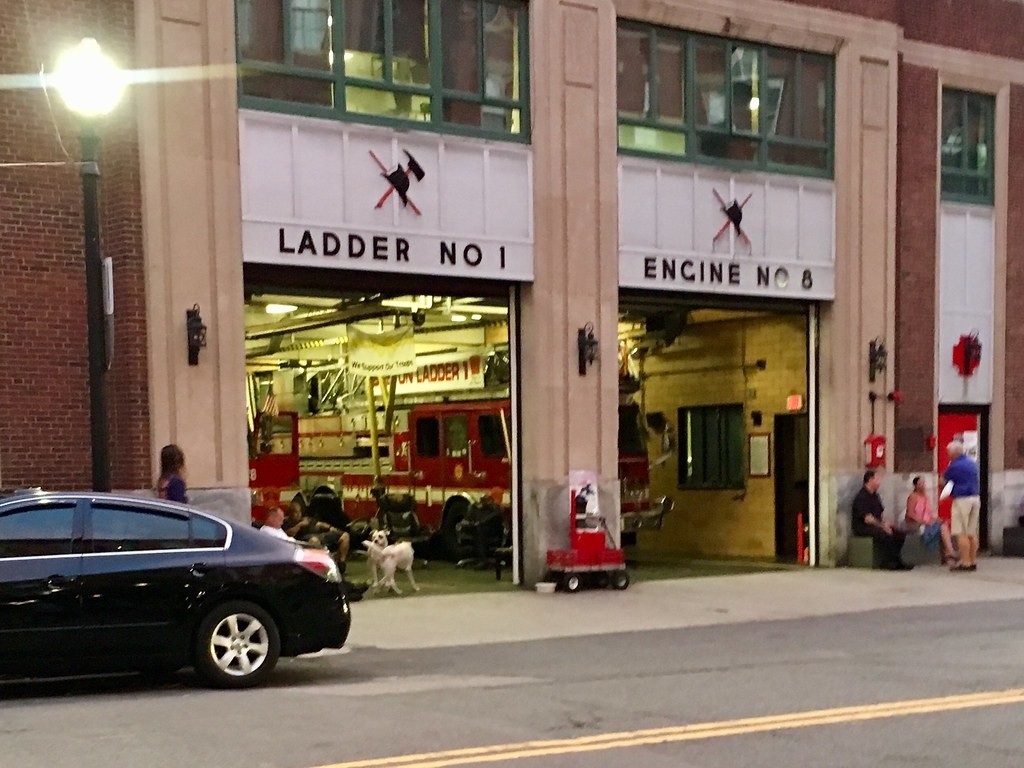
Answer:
[360, 529, 420, 596]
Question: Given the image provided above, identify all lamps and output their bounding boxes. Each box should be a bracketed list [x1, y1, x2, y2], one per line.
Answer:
[185, 302, 207, 367]
[868, 335, 889, 382]
[575, 321, 598, 375]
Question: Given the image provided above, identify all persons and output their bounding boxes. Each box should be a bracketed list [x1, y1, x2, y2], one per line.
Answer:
[851, 470, 915, 571]
[158, 445, 189, 504]
[905, 477, 959, 566]
[260, 503, 368, 602]
[939, 442, 981, 572]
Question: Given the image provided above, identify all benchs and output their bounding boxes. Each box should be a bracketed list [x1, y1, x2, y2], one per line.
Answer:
[846, 534, 938, 569]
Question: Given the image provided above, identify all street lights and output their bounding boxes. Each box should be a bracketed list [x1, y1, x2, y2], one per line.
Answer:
[47, 39, 123, 493]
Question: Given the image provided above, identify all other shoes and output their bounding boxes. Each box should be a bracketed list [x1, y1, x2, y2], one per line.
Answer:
[475, 560, 488, 570]
[944, 549, 960, 560]
[896, 558, 915, 569]
[947, 560, 962, 571]
[889, 562, 899, 570]
[343, 579, 370, 594]
[348, 592, 363, 602]
[954, 561, 971, 570]
[970, 562, 976, 570]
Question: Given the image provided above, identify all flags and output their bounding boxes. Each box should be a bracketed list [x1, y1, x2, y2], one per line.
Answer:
[261, 384, 279, 417]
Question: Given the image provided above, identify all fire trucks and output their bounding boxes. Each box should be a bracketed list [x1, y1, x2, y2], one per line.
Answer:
[246, 337, 676, 564]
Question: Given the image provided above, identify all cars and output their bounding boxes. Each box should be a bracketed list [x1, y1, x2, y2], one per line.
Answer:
[0, 489, 354, 690]
